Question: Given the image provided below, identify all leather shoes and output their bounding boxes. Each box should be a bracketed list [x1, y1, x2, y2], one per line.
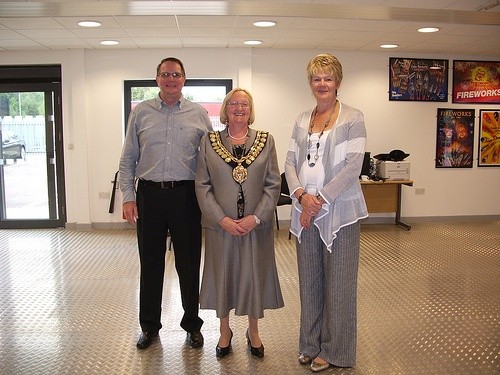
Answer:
[136, 330, 160, 348]
[186, 331, 204, 348]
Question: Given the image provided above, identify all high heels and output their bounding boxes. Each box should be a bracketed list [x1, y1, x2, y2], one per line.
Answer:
[245, 328, 265, 358]
[215, 327, 233, 358]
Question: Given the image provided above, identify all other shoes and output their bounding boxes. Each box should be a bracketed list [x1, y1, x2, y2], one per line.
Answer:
[299, 353, 311, 363]
[310, 359, 330, 372]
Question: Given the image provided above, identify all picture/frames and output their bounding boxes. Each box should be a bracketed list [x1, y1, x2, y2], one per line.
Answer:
[388, 57, 500, 169]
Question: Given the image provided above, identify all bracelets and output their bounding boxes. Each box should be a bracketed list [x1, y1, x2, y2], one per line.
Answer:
[297, 191, 307, 204]
[316, 194, 325, 203]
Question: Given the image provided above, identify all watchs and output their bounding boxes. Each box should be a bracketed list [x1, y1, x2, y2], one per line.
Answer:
[253, 214, 263, 226]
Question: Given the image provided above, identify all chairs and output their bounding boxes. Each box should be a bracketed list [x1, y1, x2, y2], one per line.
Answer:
[1, 131, 27, 165]
[275, 172, 293, 239]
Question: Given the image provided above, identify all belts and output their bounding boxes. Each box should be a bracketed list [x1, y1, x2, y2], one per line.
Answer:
[139, 177, 192, 189]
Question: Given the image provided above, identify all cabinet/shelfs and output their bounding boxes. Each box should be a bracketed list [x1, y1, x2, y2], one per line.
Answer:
[376, 160, 410, 180]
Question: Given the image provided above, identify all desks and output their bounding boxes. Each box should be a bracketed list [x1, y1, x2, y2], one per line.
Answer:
[359, 179, 413, 231]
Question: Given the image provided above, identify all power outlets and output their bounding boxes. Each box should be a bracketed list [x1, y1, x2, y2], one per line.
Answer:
[99, 192, 110, 198]
[415, 188, 425, 194]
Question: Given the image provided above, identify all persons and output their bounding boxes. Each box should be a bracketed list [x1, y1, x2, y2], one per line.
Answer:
[118, 57, 215, 351]
[283, 53, 368, 372]
[195, 87, 285, 359]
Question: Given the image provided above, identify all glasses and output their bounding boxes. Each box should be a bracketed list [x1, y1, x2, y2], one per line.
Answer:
[158, 72, 183, 79]
[228, 100, 250, 108]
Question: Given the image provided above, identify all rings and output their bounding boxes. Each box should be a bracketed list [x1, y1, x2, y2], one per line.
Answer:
[310, 210, 314, 215]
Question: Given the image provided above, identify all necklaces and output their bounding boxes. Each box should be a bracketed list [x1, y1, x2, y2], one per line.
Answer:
[226, 127, 248, 140]
[230, 140, 248, 184]
[304, 100, 338, 167]
